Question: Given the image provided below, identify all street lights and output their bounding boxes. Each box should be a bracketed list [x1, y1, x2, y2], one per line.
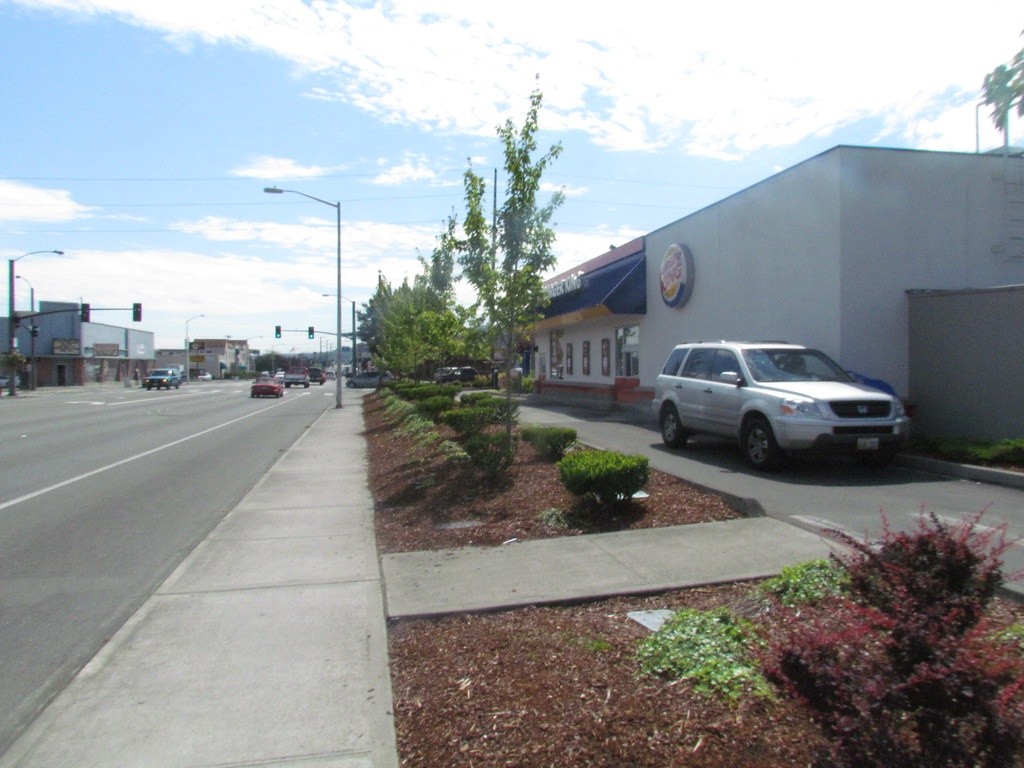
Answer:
[272, 343, 284, 373]
[7, 250, 64, 393]
[246, 336, 263, 371]
[264, 186, 342, 407]
[16, 275, 36, 389]
[322, 294, 356, 373]
[185, 314, 205, 385]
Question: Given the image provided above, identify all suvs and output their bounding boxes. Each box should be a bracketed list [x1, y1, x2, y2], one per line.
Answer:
[652, 338, 911, 471]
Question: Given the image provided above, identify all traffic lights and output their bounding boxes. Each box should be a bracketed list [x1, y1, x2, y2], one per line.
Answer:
[275, 326, 281, 338]
[133, 303, 142, 321]
[308, 327, 314, 339]
[80, 303, 90, 321]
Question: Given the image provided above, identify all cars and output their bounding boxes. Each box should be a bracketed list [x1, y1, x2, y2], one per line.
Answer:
[274, 372, 285, 383]
[436, 367, 476, 383]
[261, 370, 270, 377]
[346, 371, 392, 387]
[284, 365, 311, 388]
[325, 371, 336, 380]
[251, 377, 284, 397]
[198, 372, 212, 381]
[143, 368, 181, 390]
[308, 367, 326, 384]
